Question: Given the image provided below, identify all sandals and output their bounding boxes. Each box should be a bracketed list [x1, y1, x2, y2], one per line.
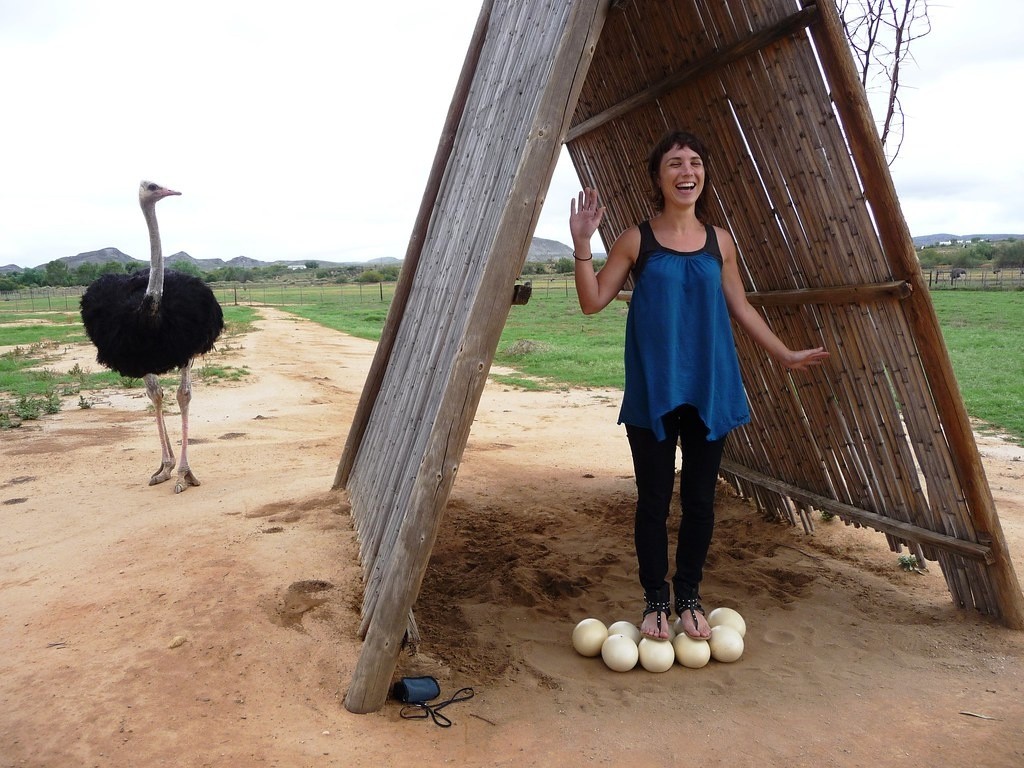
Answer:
[640, 609, 671, 641]
[675, 603, 713, 641]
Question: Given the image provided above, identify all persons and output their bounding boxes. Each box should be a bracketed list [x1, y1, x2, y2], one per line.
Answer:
[570, 131, 829, 641]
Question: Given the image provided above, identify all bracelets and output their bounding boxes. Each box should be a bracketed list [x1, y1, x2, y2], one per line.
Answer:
[573, 251, 592, 261]
